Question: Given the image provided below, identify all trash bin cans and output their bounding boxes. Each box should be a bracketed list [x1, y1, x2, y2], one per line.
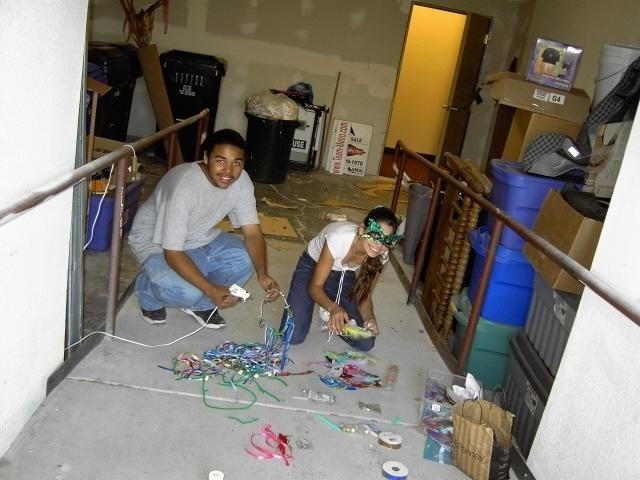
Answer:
[86, 41, 226, 163]
[244, 112, 301, 184]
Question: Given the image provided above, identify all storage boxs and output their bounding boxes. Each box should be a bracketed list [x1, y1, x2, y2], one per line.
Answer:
[447, 285, 520, 394]
[86, 171, 146, 252]
[520, 185, 603, 296]
[521, 271, 579, 377]
[482, 156, 588, 250]
[492, 328, 555, 459]
[466, 224, 537, 328]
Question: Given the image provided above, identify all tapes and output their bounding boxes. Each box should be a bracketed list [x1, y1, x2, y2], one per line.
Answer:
[378, 432, 402, 449]
[383, 461, 409, 480]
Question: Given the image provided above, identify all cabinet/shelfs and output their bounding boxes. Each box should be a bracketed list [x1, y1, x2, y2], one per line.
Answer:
[484, 71, 592, 163]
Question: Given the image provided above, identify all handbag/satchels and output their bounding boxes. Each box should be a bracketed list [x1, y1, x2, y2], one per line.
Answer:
[452, 399, 515, 480]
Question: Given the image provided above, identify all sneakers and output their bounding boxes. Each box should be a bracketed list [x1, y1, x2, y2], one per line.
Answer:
[179, 307, 228, 329]
[139, 305, 167, 324]
[319, 306, 331, 322]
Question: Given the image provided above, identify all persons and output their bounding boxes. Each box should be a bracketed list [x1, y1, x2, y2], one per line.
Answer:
[279, 205, 400, 354]
[127, 128, 282, 332]
